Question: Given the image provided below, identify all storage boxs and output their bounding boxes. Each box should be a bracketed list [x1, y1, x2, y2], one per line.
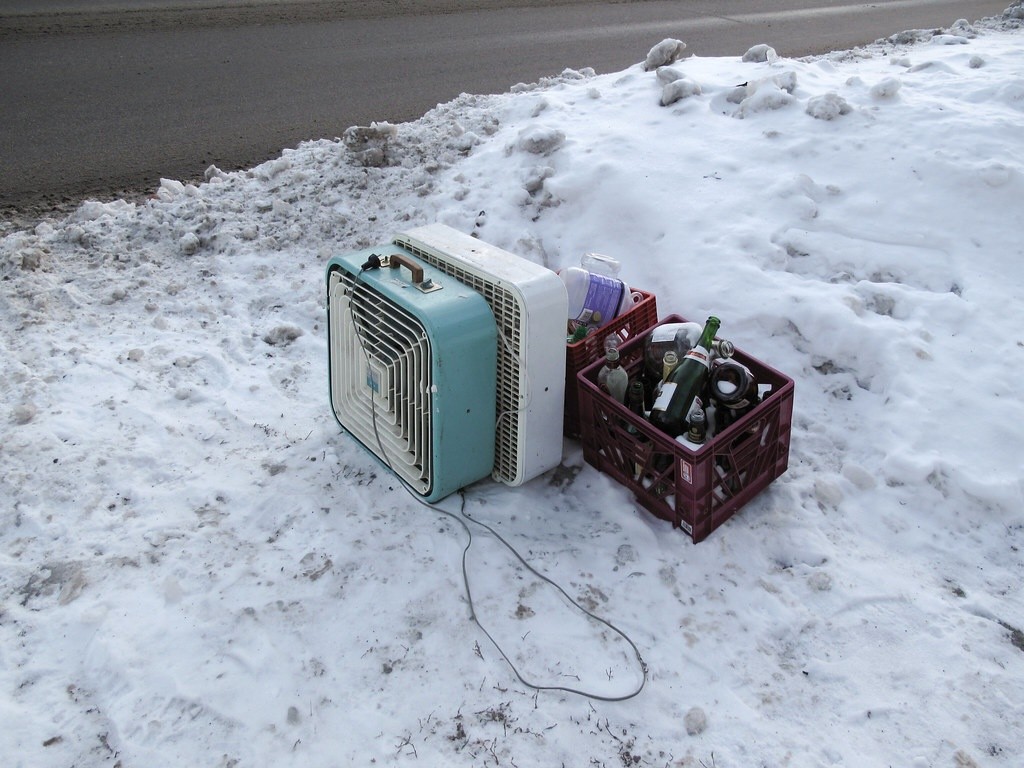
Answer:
[577, 313, 795, 547]
[564, 272, 658, 450]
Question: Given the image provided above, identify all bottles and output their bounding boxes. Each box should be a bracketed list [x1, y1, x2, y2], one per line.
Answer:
[558, 252, 775, 513]
